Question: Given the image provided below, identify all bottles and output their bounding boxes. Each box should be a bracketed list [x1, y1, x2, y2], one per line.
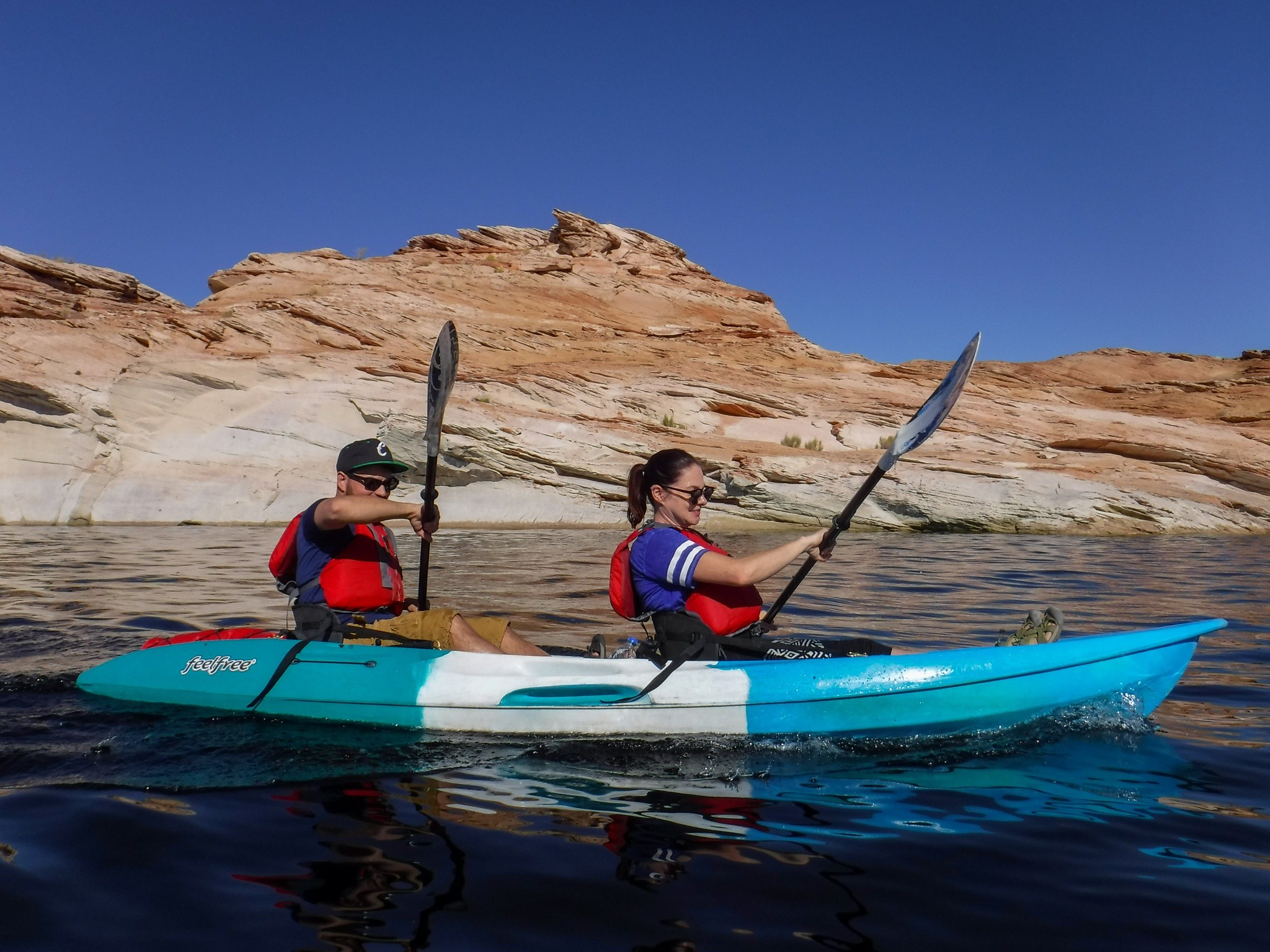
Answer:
[608, 637, 638, 661]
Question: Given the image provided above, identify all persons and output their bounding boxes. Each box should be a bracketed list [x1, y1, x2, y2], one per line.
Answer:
[295, 439, 552, 656]
[626, 450, 1064, 662]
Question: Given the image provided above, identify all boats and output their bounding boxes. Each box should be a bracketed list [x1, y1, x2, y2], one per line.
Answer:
[77, 615, 1232, 746]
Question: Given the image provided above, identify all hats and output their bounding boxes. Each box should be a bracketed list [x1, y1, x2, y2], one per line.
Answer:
[335, 436, 411, 473]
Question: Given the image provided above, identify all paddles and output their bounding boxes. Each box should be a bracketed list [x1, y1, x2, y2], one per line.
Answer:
[419, 316, 463, 611]
[760, 331, 982, 632]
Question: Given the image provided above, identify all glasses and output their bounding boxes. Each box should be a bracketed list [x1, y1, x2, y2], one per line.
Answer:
[346, 470, 400, 494]
[657, 484, 717, 503]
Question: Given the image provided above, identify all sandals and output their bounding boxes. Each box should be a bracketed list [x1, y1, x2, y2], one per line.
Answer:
[1005, 606, 1063, 645]
[584, 634, 607, 659]
